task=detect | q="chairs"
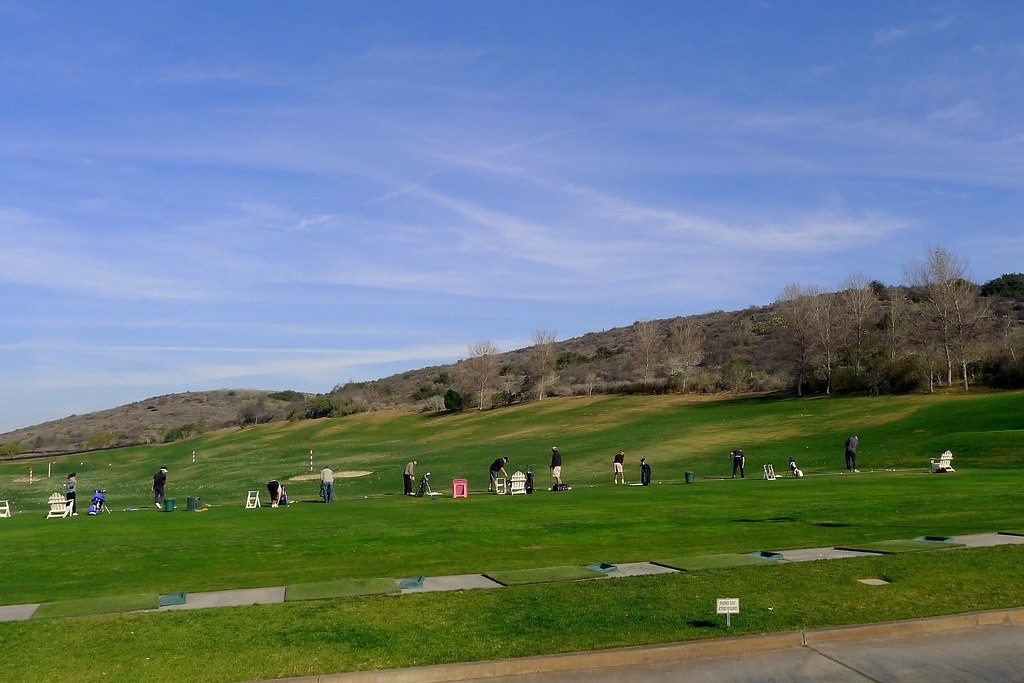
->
[930,450,956,472]
[510,470,527,496]
[763,464,776,481]
[246,490,261,508]
[47,492,74,519]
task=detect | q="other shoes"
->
[271,504,279,508]
[72,512,78,516]
[732,474,736,478]
[155,502,162,509]
[848,470,853,473]
[854,469,860,473]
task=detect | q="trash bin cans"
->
[685,471,694,484]
[164,498,174,511]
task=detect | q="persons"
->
[549,447,562,485]
[490,457,509,492]
[267,480,282,508]
[66,473,78,516]
[845,436,860,472]
[152,467,168,508]
[404,460,418,495]
[730,448,745,478]
[320,467,333,502]
[613,451,625,484]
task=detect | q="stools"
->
[0,500,12,518]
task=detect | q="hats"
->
[620,451,625,455]
[549,446,558,451]
[504,457,510,463]
[855,434,860,443]
[160,467,169,473]
